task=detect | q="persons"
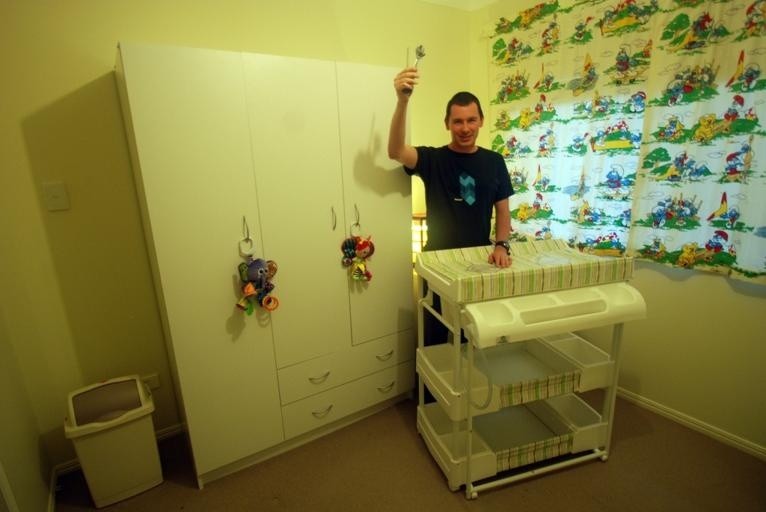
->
[385,66,515,402]
[338,233,377,282]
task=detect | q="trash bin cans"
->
[63,375,164,508]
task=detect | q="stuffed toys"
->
[236,256,279,326]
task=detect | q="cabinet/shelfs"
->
[410,238,649,500]
[113,39,422,494]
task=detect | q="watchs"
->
[495,239,513,256]
